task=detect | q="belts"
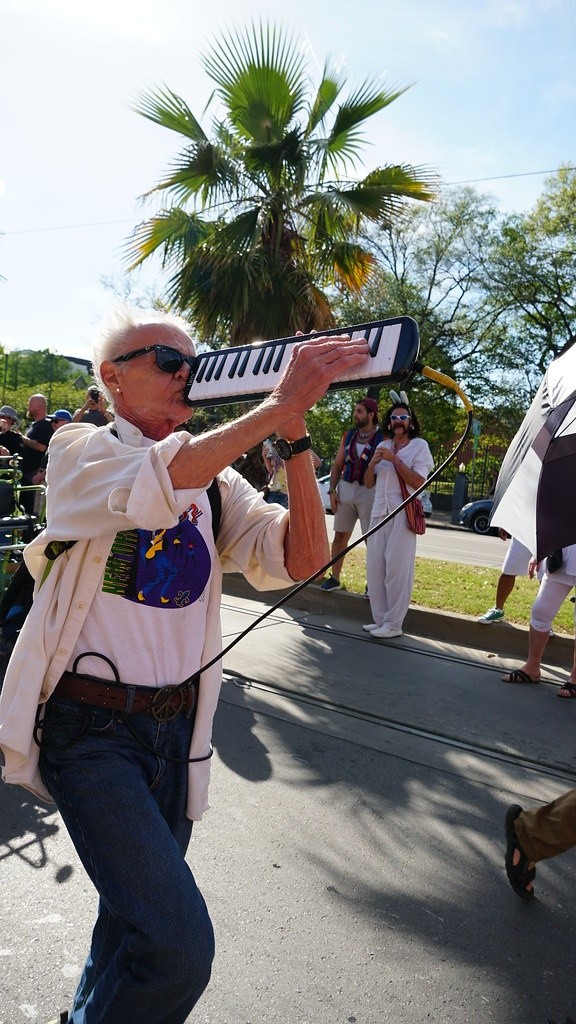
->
[58,676,202,715]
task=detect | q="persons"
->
[33,410,73,512]
[0,308,370,1024]
[500,544,576,697]
[363,389,435,638]
[478,527,554,636]
[15,394,53,514]
[320,399,389,599]
[72,385,116,428]
[0,405,24,456]
[262,448,321,510]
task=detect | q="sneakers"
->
[321,573,341,591]
[370,625,403,638]
[363,585,370,599]
[363,624,381,631]
[477,608,505,624]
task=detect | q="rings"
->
[381,453,382,457]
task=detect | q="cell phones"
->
[91,389,99,403]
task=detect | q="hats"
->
[46,410,73,422]
[360,398,378,414]
[1,406,21,425]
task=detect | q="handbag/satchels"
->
[404,492,425,535]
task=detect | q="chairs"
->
[0,480,38,526]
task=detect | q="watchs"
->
[273,434,312,460]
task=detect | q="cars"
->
[317,472,432,518]
[459,499,510,535]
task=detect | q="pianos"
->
[181,312,422,408]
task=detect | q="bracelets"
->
[327,490,336,495]
[104,413,108,416]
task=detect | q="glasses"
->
[390,415,410,421]
[114,345,196,373]
[51,418,65,424]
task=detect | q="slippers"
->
[506,804,537,898]
[554,681,576,699]
[502,669,542,684]
